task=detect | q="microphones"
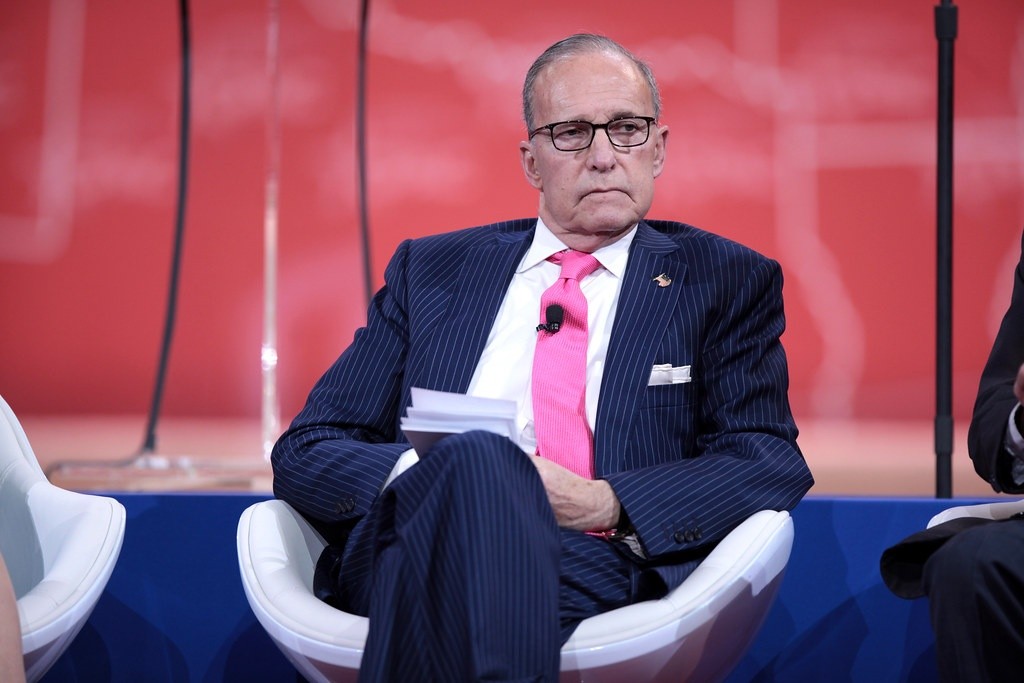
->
[536,305,564,333]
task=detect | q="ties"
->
[530,250,615,540]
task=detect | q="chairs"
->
[0,394,126,683]
[236,498,796,683]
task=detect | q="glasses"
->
[527,116,659,152]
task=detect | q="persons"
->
[271,33,816,683]
[882,231,1024,683]
